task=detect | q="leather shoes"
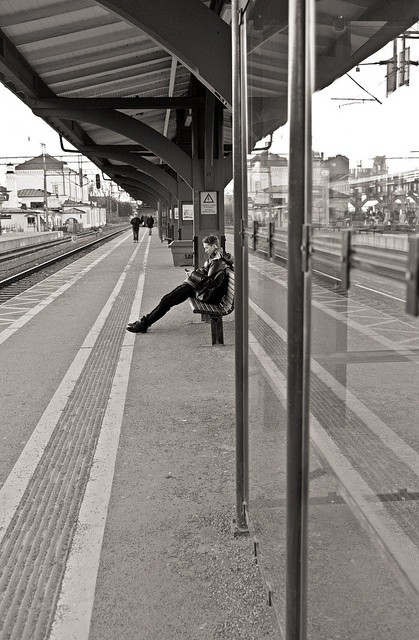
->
[128,321,139,326]
[126,322,147,333]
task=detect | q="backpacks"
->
[195,266,228,308]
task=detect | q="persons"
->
[126,235,229,334]
[130,213,154,243]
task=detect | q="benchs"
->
[187,268,235,346]
